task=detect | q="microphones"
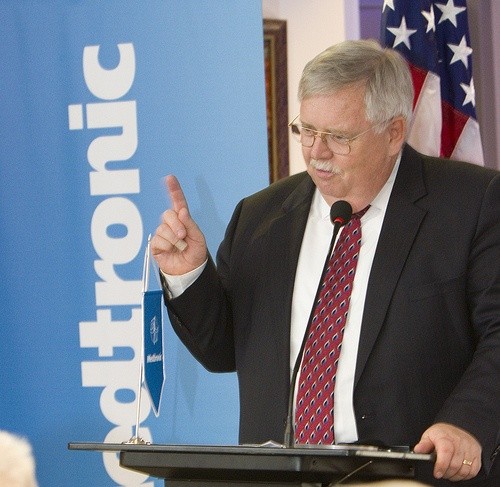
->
[284,200,353,449]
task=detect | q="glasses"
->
[289,113,386,156]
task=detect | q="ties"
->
[294,205,371,445]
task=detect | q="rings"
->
[462,459,473,465]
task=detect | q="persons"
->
[148,39,500,483]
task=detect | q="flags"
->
[143,291,166,418]
[382,0,486,168]
